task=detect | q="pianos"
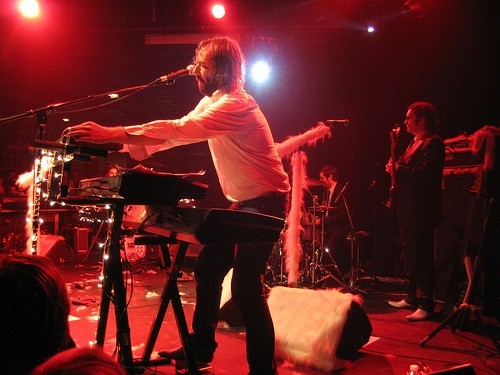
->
[119,200,288,247]
[77,166,210,204]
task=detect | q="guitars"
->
[387,126,403,207]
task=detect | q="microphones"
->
[158,64,195,83]
[334,180,350,204]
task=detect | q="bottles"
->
[407,364,419,375]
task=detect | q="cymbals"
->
[306,175,327,188]
[311,204,338,211]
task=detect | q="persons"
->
[105,165,118,177]
[0,251,127,375]
[306,165,352,293]
[66,38,291,375]
[1,170,32,198]
[385,102,446,321]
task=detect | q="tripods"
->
[418,189,500,354]
[297,194,369,295]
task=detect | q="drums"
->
[301,213,325,242]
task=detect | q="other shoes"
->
[388,299,416,308]
[406,309,433,321]
[158,345,185,360]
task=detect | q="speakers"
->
[22,234,75,265]
[429,166,500,315]
[266,285,372,360]
